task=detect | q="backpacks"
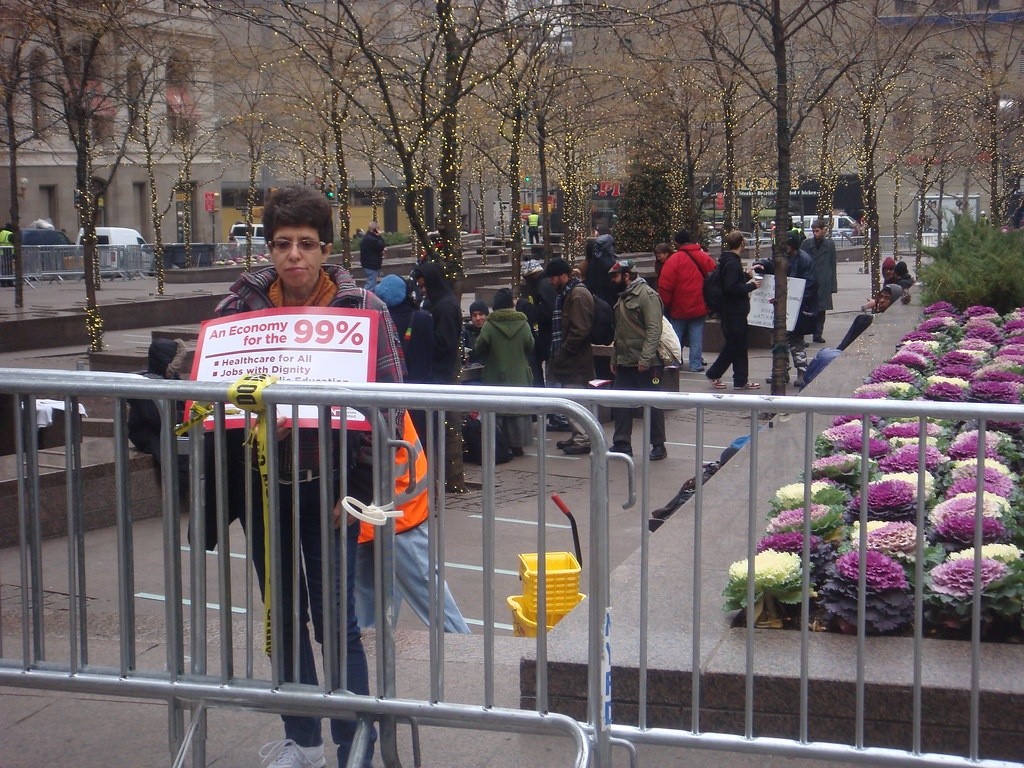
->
[577,285,614,346]
[703,257,739,313]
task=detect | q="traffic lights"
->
[519,176,529,182]
[328,182,335,201]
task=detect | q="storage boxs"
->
[507,552,585,637]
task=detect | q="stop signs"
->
[521,209,532,219]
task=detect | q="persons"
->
[125,338,189,484]
[213,183,407,768]
[351,208,914,462]
[979,211,989,226]
[0,222,20,287]
[353,408,470,635]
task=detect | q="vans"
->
[792,215,863,243]
[76,227,152,274]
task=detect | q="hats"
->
[674,230,691,243]
[788,230,802,249]
[538,257,570,279]
[608,259,638,274]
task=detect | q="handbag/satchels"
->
[658,314,682,366]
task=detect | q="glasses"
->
[270,238,325,252]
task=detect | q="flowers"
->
[725,300,1024,632]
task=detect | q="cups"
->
[755,276,763,282]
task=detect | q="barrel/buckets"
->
[507,593,586,637]
[519,551,581,624]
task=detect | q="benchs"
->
[0,240,873,548]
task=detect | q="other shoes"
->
[734,379,761,390]
[547,421,571,431]
[793,369,806,386]
[555,435,592,455]
[813,334,826,343]
[705,372,726,388]
[607,444,634,458]
[650,445,668,461]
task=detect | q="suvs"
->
[21,229,76,267]
[227,224,266,252]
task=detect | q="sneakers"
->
[267,743,327,768]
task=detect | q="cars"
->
[709,231,771,247]
